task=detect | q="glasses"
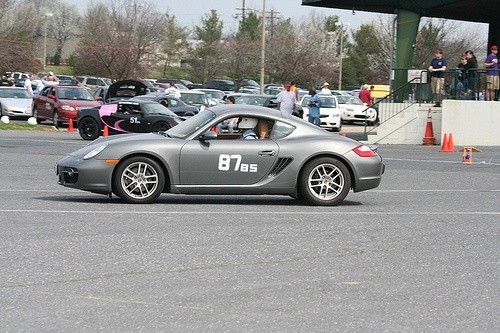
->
[462,58,467,60]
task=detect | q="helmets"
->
[238,118,259,129]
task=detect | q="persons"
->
[309,88,321,126]
[484,45,500,101]
[359,84,375,105]
[321,83,331,94]
[451,50,485,101]
[37,73,59,94]
[25,74,34,95]
[241,120,274,139]
[165,81,181,104]
[277,82,296,115]
[290,81,299,101]
[219,96,238,133]
[0,75,13,86]
[429,49,447,107]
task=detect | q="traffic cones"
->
[67,119,75,133]
[102,125,109,137]
[446,133,456,152]
[422,108,436,146]
[439,133,449,153]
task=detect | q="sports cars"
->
[54,102,385,206]
[76,98,188,141]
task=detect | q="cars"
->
[0,70,392,140]
[30,84,101,127]
[0,85,35,119]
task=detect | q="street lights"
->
[335,17,350,91]
[43,12,54,72]
